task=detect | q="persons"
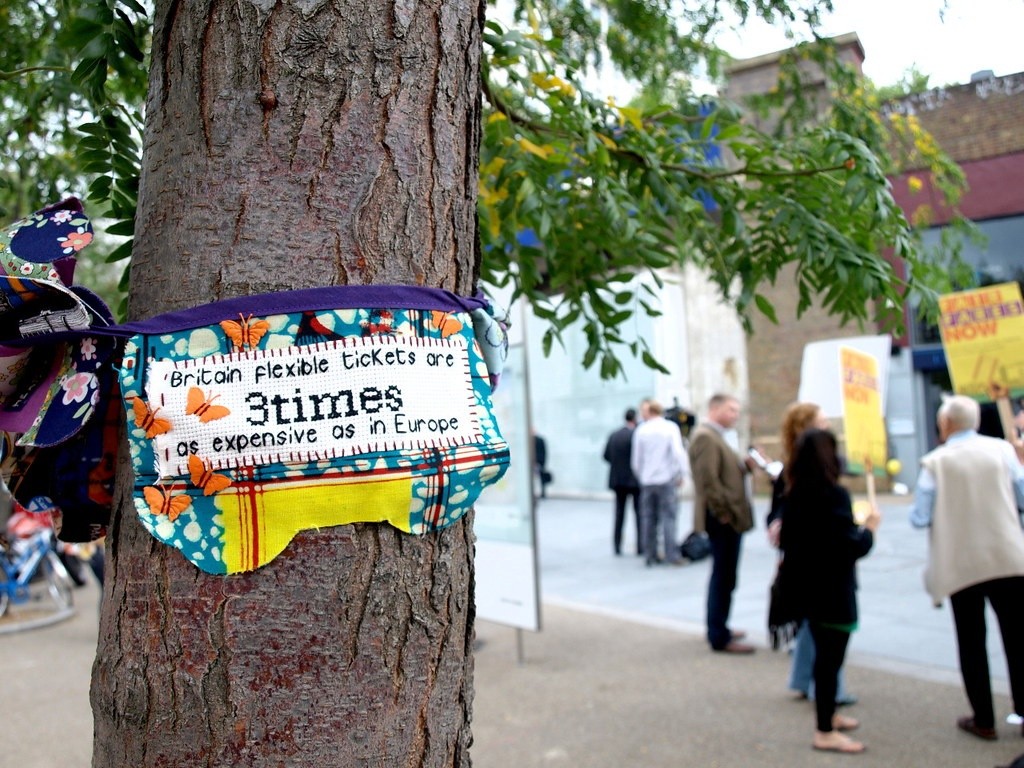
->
[637,397,651,424]
[533,431,546,498]
[631,402,690,567]
[773,405,856,707]
[909,396,1024,738]
[690,395,760,654]
[780,429,878,755]
[604,409,641,555]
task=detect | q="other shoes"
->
[647,557,666,565]
[831,713,859,729]
[813,730,867,753]
[835,693,857,705]
[672,556,690,565]
[728,629,746,639]
[723,641,753,653]
[960,715,998,739]
